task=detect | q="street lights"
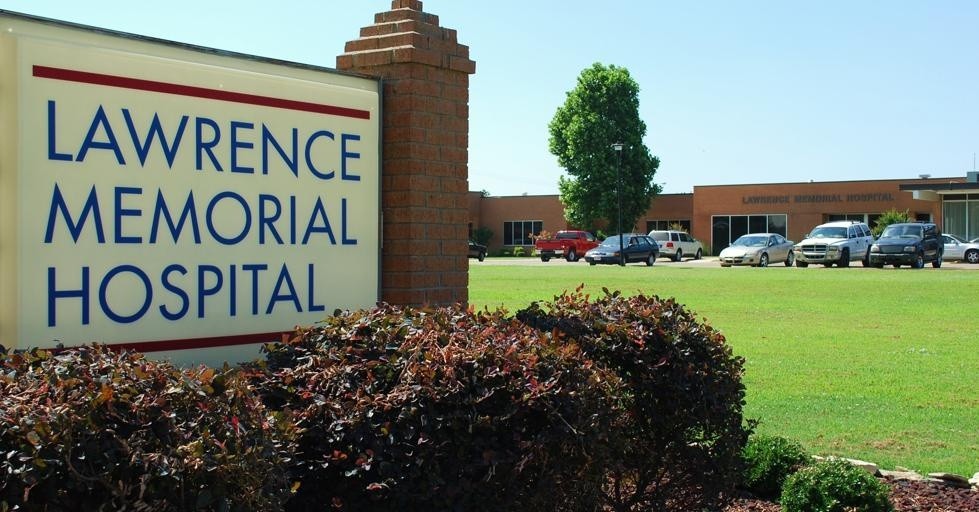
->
[612,140,625,266]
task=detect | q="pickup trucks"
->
[534,230,603,262]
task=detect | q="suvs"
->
[793,220,944,267]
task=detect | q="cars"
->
[584,230,703,266]
[942,220,979,268]
[719,232,795,267]
[468,237,488,261]
